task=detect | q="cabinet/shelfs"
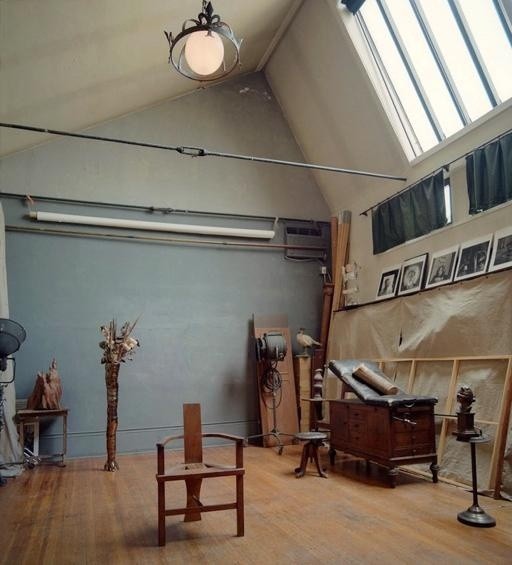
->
[326,397,439,488]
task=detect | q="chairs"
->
[156,402,245,547]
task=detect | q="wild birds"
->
[296,328,322,354]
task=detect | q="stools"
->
[295,432,328,479]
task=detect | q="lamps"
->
[162,0,245,90]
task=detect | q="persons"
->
[498,241,512,261]
[431,266,449,282]
[404,266,420,289]
[383,279,393,295]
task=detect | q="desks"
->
[16,408,68,468]
